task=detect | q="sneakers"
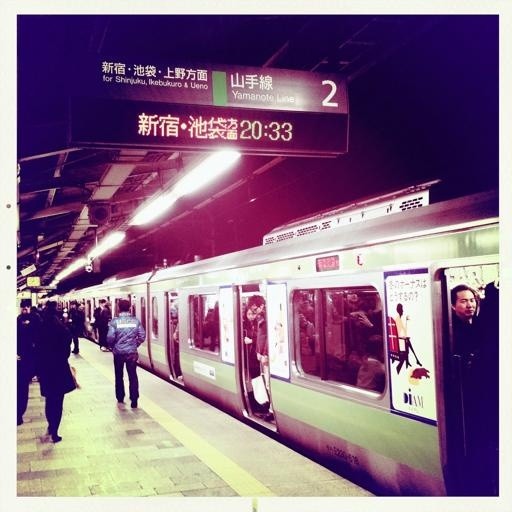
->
[264,414,274,423]
[72,348,79,354]
[116,396,138,409]
[52,434,62,443]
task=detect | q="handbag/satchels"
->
[251,372,269,405]
[63,363,80,394]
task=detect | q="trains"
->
[37,179,499,496]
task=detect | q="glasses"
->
[252,306,260,313]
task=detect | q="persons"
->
[17,298,86,441]
[447,281,499,472]
[171,304,183,380]
[241,295,275,422]
[193,298,219,352]
[92,299,146,409]
[297,286,385,394]
